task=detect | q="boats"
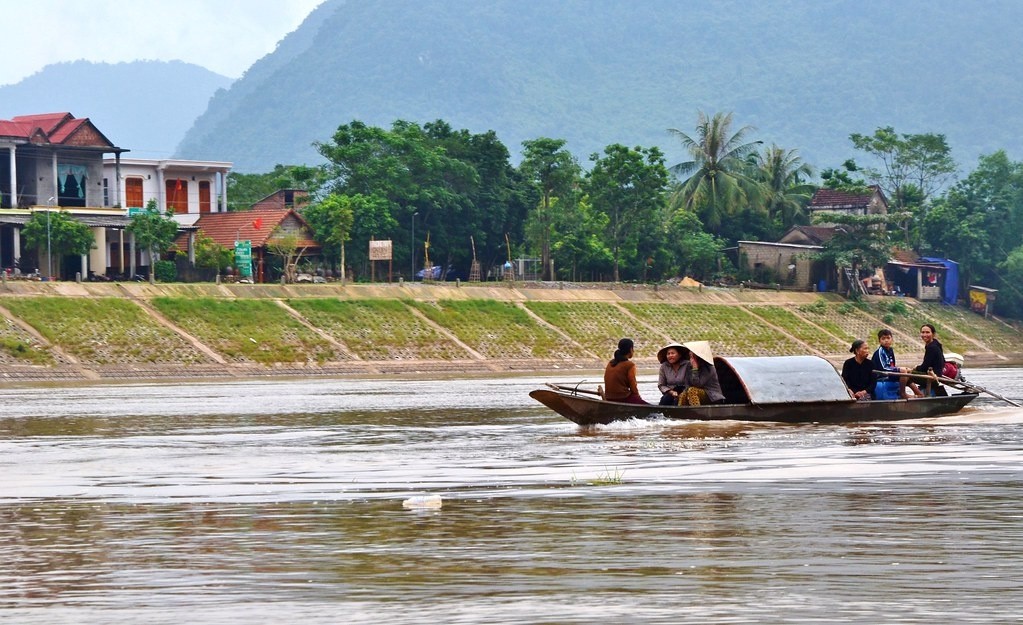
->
[528,355,981,424]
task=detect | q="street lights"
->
[412,211,419,282]
[47,196,55,281]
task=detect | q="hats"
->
[657,341,691,364]
[683,340,715,366]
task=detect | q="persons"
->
[842,341,878,401]
[603,339,649,403]
[872,330,925,398]
[911,324,945,388]
[658,341,723,407]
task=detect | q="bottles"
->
[402,494,442,509]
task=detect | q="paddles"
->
[545,382,600,396]
[872,369,965,385]
[942,374,1021,407]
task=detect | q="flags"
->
[175,177,181,190]
